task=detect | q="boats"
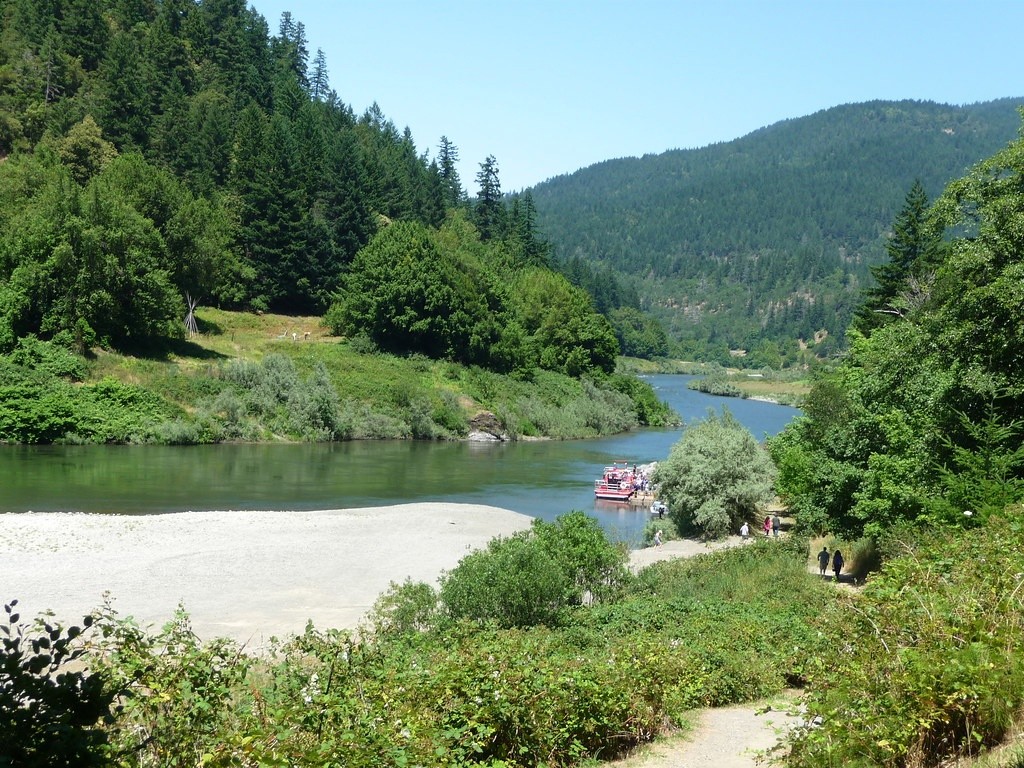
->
[594,461,642,499]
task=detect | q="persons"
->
[763,516,770,535]
[832,550,844,579]
[659,508,664,519]
[740,522,749,540]
[772,514,780,535]
[653,530,662,549]
[817,547,829,578]
[633,464,636,474]
[643,478,648,490]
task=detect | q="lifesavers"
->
[620,482,626,489]
